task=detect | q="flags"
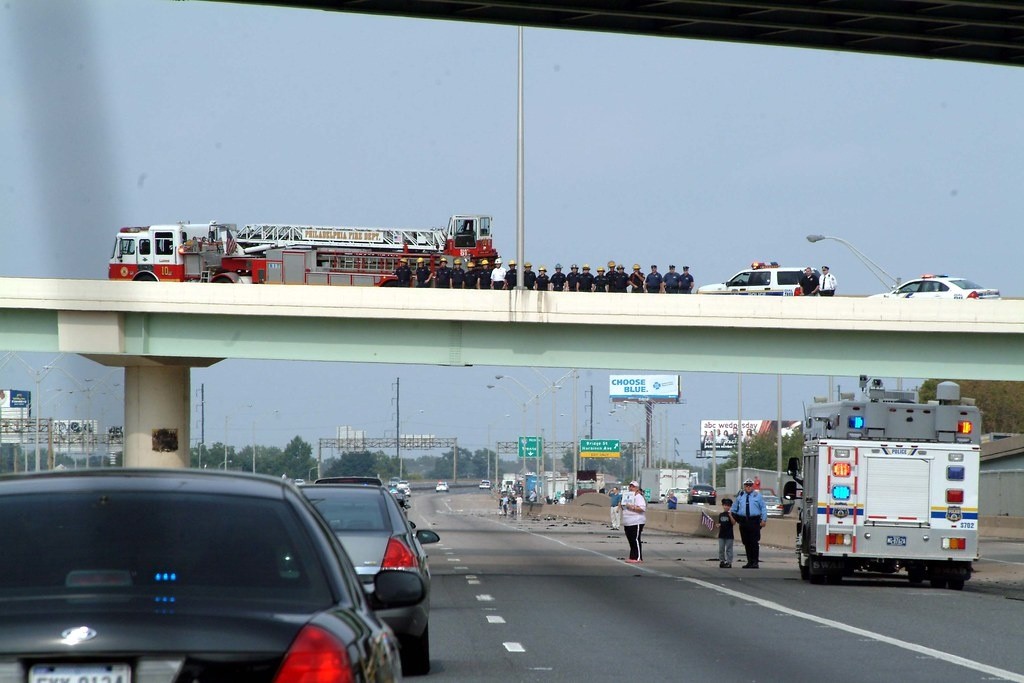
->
[701,513,714,531]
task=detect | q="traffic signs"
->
[518,436,543,459]
[579,439,621,458]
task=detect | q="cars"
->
[479,480,491,491]
[0,468,428,683]
[733,487,795,518]
[864,273,1001,299]
[285,482,441,678]
[658,485,718,505]
[435,482,450,492]
[386,476,413,520]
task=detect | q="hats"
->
[822,266,829,270]
[651,265,690,269]
[630,481,639,487]
[743,478,754,485]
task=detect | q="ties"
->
[823,278,825,289]
[746,494,749,519]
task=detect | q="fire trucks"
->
[108,215,499,288]
[783,375,983,589]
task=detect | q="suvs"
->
[696,262,822,297]
[311,473,386,530]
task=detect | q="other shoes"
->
[720,561,731,568]
[625,559,638,563]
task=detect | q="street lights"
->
[308,466,318,484]
[487,384,530,507]
[224,400,254,471]
[218,459,232,470]
[494,374,545,505]
[0,349,125,472]
[199,440,216,469]
[252,409,280,473]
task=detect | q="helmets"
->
[509,260,516,266]
[597,267,606,272]
[571,264,578,269]
[467,262,475,268]
[494,259,502,264]
[634,264,641,269]
[608,262,616,267]
[617,265,625,269]
[538,267,545,271]
[583,264,590,270]
[416,258,424,263]
[440,258,448,264]
[524,263,532,267]
[555,264,561,268]
[400,258,408,263]
[481,260,489,264]
[454,260,462,265]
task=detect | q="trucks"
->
[639,467,690,503]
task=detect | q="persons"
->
[434,258,518,290]
[512,495,523,514]
[667,492,677,509]
[396,258,413,287]
[416,257,433,288]
[800,268,819,296]
[716,498,736,569]
[730,478,767,569]
[529,491,537,502]
[819,266,837,296]
[500,496,509,514]
[524,263,695,294]
[618,481,647,563]
[608,487,622,530]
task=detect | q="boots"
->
[742,551,759,568]
